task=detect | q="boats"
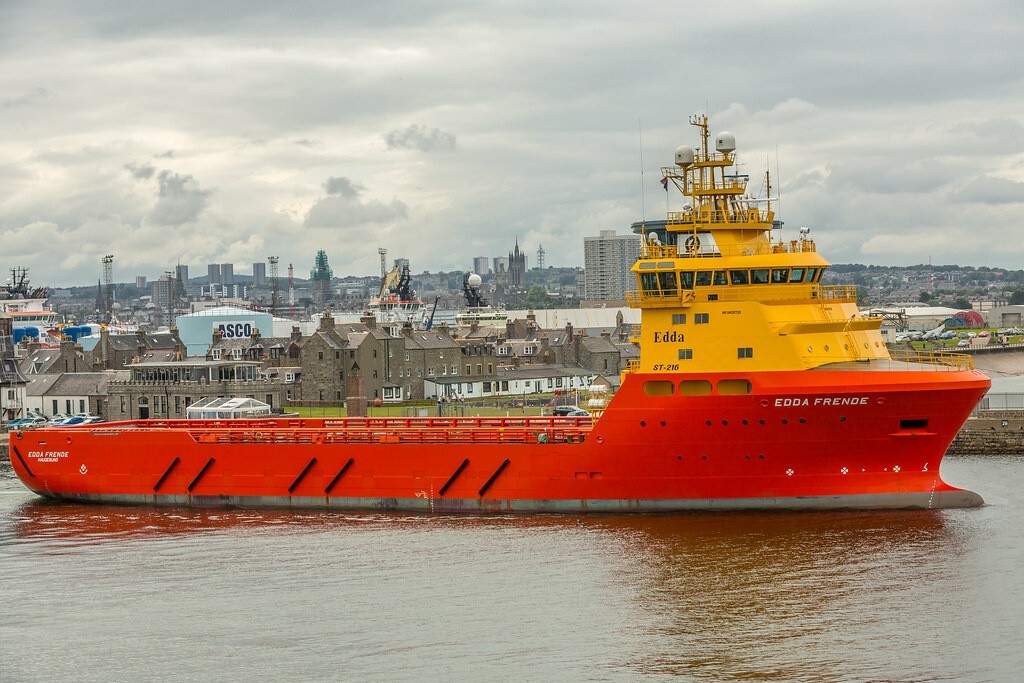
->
[8,112,992,515]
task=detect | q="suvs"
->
[553,405,580,416]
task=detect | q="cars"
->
[567,410,589,416]
[8,411,101,431]
[895,328,1024,348]
